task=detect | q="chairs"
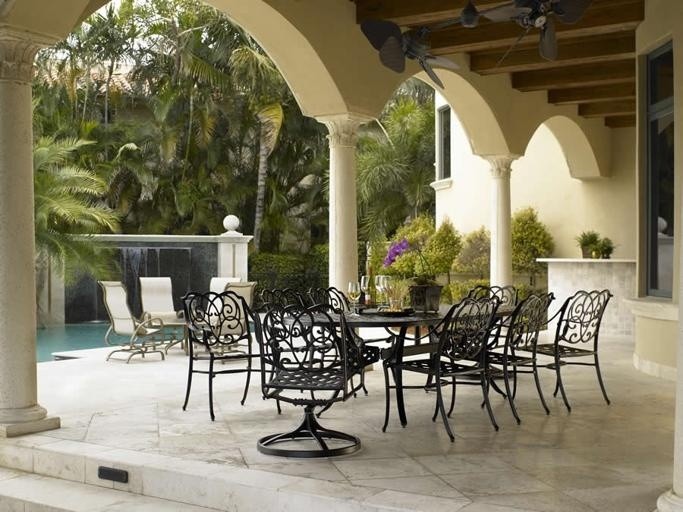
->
[97,276,259,365]
[511,289,613,414]
[182,291,263,420]
[257,285,553,457]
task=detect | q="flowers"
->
[381,236,436,284]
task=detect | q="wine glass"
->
[346,280,361,319]
[360,276,395,306]
